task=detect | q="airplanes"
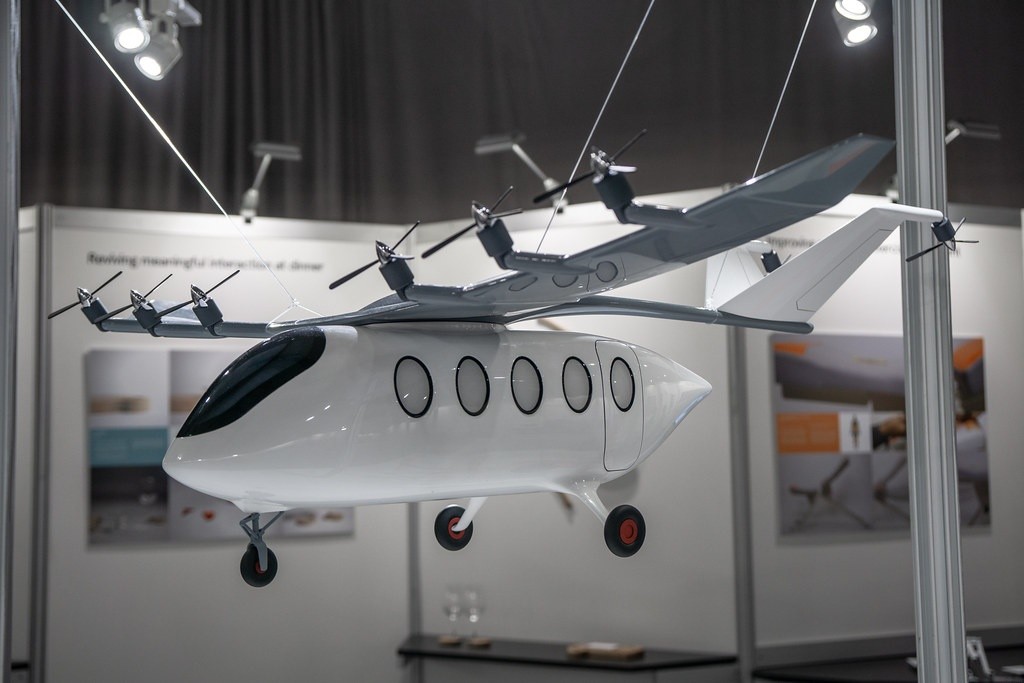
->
[47,126,977,588]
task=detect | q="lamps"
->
[830,0,878,47]
[99,0,203,80]
